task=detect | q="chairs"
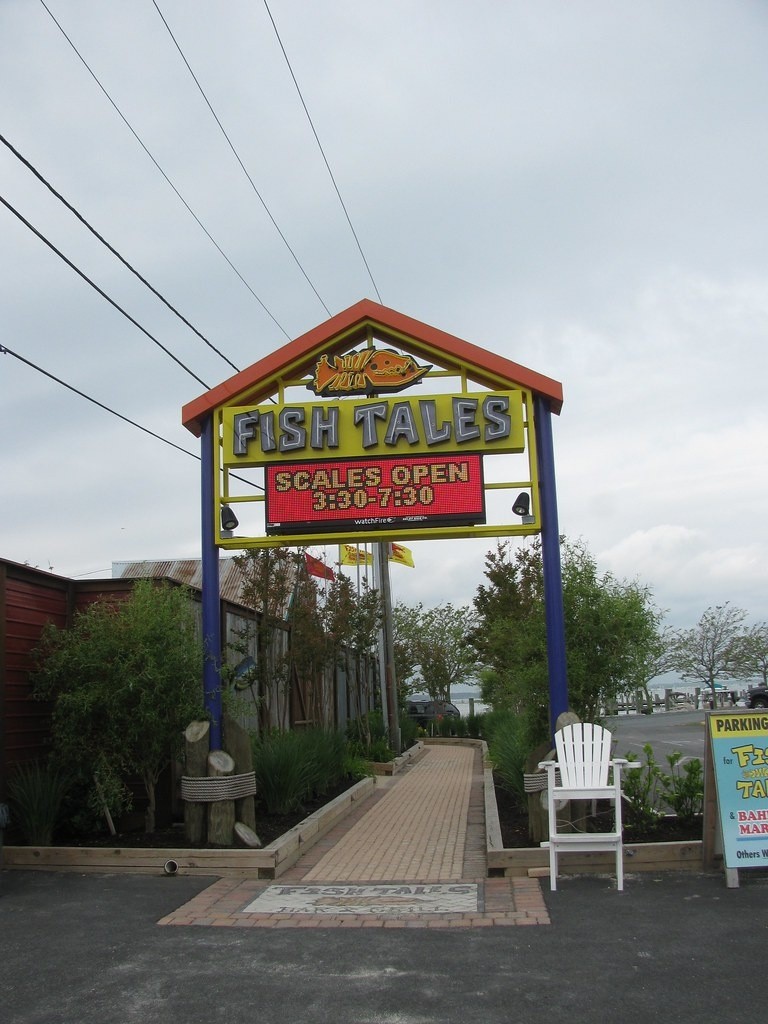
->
[538,723,622,892]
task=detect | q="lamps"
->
[232,656,258,690]
[511,492,533,519]
[220,506,239,530]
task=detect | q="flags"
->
[306,553,335,582]
[340,544,372,566]
[387,542,414,568]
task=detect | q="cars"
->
[405,700,460,732]
[745,686,768,711]
